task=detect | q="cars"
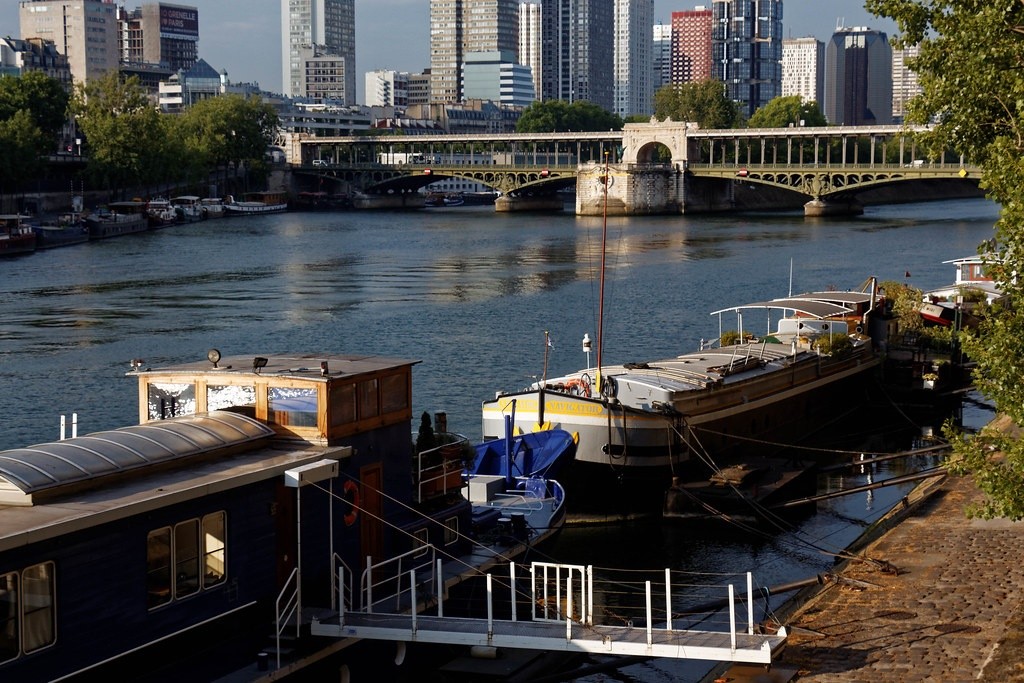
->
[904,160,930,168]
[428,155,441,164]
[303,160,312,166]
[409,156,430,164]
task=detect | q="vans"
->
[313,160,328,167]
[23,197,64,215]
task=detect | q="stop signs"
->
[542,169,548,176]
[424,168,431,175]
[739,168,748,176]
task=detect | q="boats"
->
[481,256,896,498]
[225,191,288,211]
[298,192,329,210]
[0,349,581,683]
[146,197,178,225]
[425,194,464,207]
[202,198,227,218]
[169,196,208,224]
[88,201,148,238]
[32,212,90,243]
[0,213,37,255]
[916,252,1018,310]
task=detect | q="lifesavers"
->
[344,480,358,524]
[562,378,592,403]
